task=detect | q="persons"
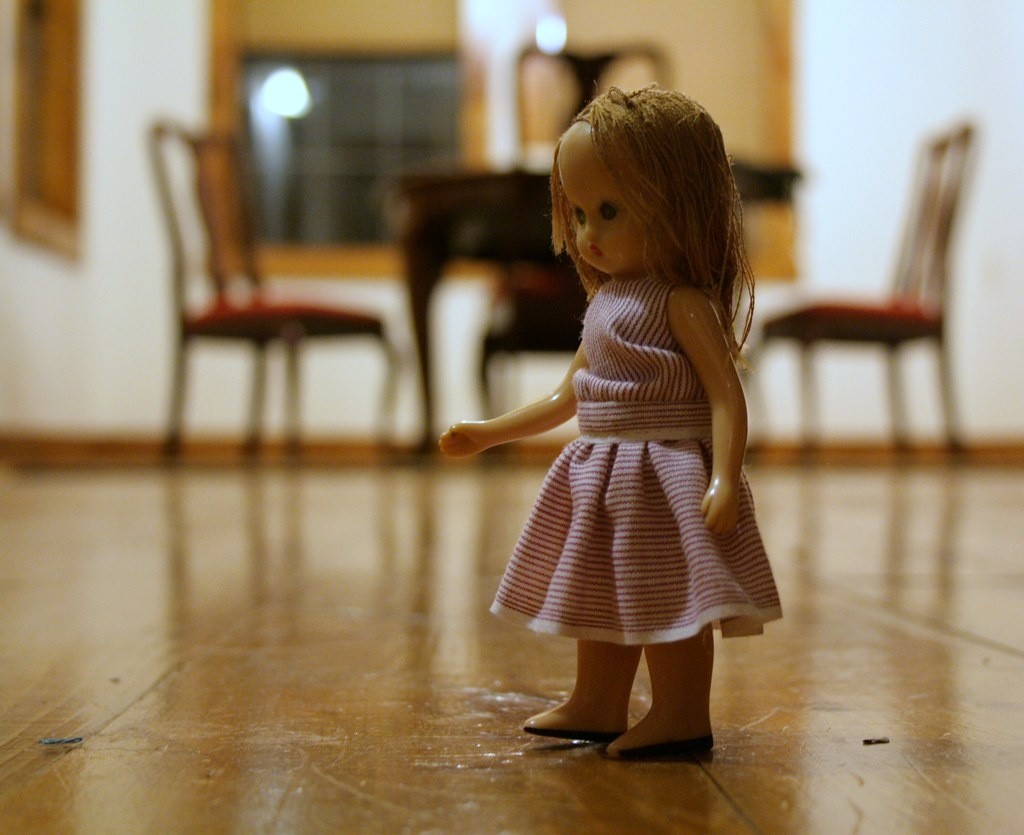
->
[437,88,785,761]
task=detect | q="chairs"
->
[742,124,975,469]
[150,121,405,468]
[473,42,668,422]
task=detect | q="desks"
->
[393,167,590,451]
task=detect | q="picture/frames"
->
[8,1,86,259]
